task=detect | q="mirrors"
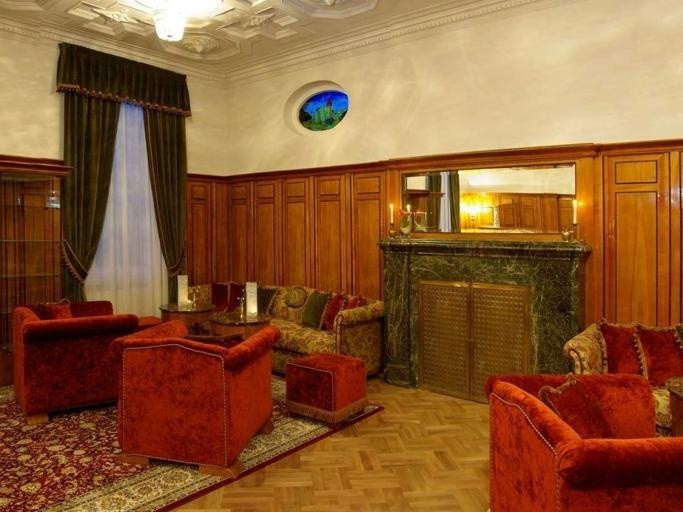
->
[401,162,577,236]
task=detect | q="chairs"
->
[480,316,683,511]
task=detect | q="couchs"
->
[183,278,383,381]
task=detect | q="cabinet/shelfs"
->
[495,191,573,234]
[404,188,445,233]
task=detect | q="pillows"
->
[207,279,278,336]
[299,288,366,334]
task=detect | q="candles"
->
[388,203,395,223]
[572,198,578,226]
[406,201,412,224]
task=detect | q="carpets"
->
[0,375,385,512]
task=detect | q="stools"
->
[283,348,369,428]
